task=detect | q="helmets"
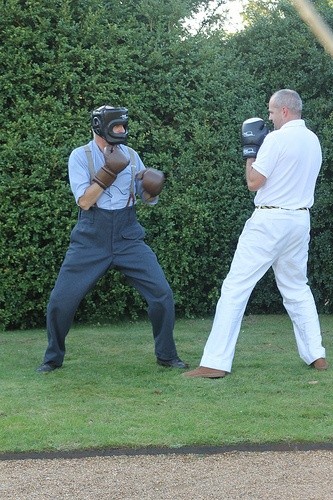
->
[91,108,129,145]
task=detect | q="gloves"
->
[240,117,266,158]
[92,148,129,191]
[137,167,165,195]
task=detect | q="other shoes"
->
[155,360,187,370]
[313,359,328,370]
[178,367,226,379]
[36,363,54,372]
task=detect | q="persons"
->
[35,105,190,372]
[178,89,326,377]
